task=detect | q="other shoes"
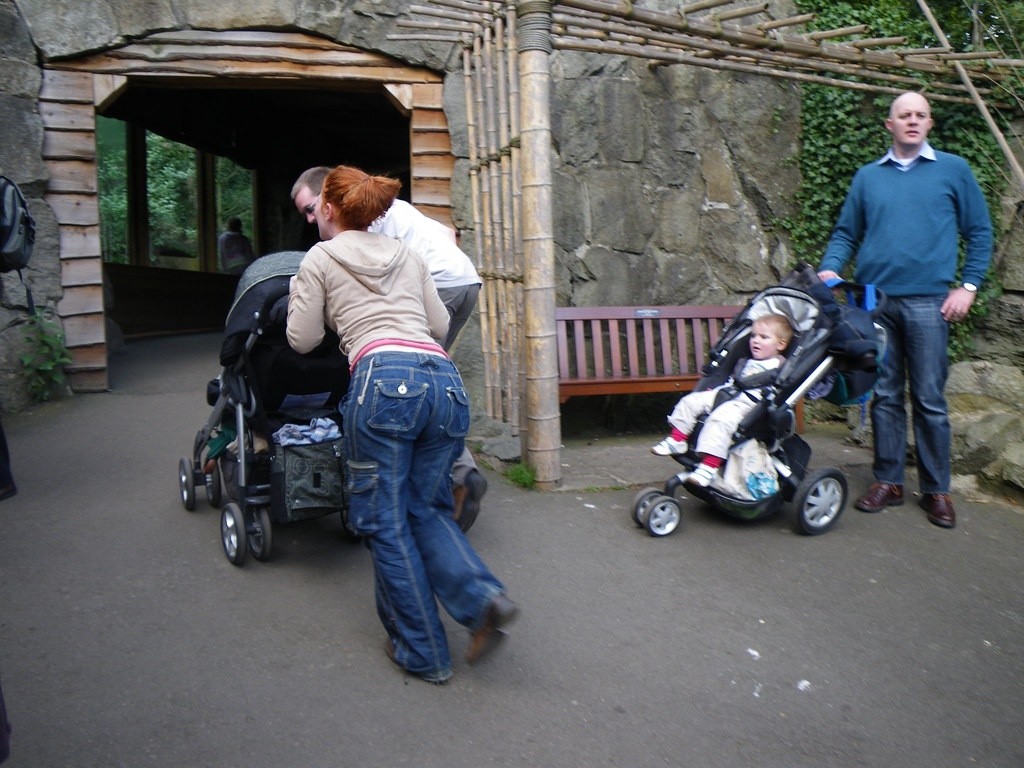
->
[452,469,487,535]
[466,594,516,665]
[0,480,16,501]
[385,635,399,663]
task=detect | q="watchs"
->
[962,282,977,291]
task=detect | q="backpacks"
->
[0,176,36,273]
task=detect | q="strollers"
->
[180,254,365,568]
[631,265,889,540]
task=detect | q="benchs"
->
[554,304,804,434]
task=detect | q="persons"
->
[649,313,794,487]
[219,164,523,685]
[0,422,19,502]
[816,89,992,528]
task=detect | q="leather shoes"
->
[854,482,904,512]
[918,492,956,527]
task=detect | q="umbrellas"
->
[202,417,237,474]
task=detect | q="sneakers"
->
[650,435,688,456]
[687,462,720,488]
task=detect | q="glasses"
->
[302,193,321,218]
